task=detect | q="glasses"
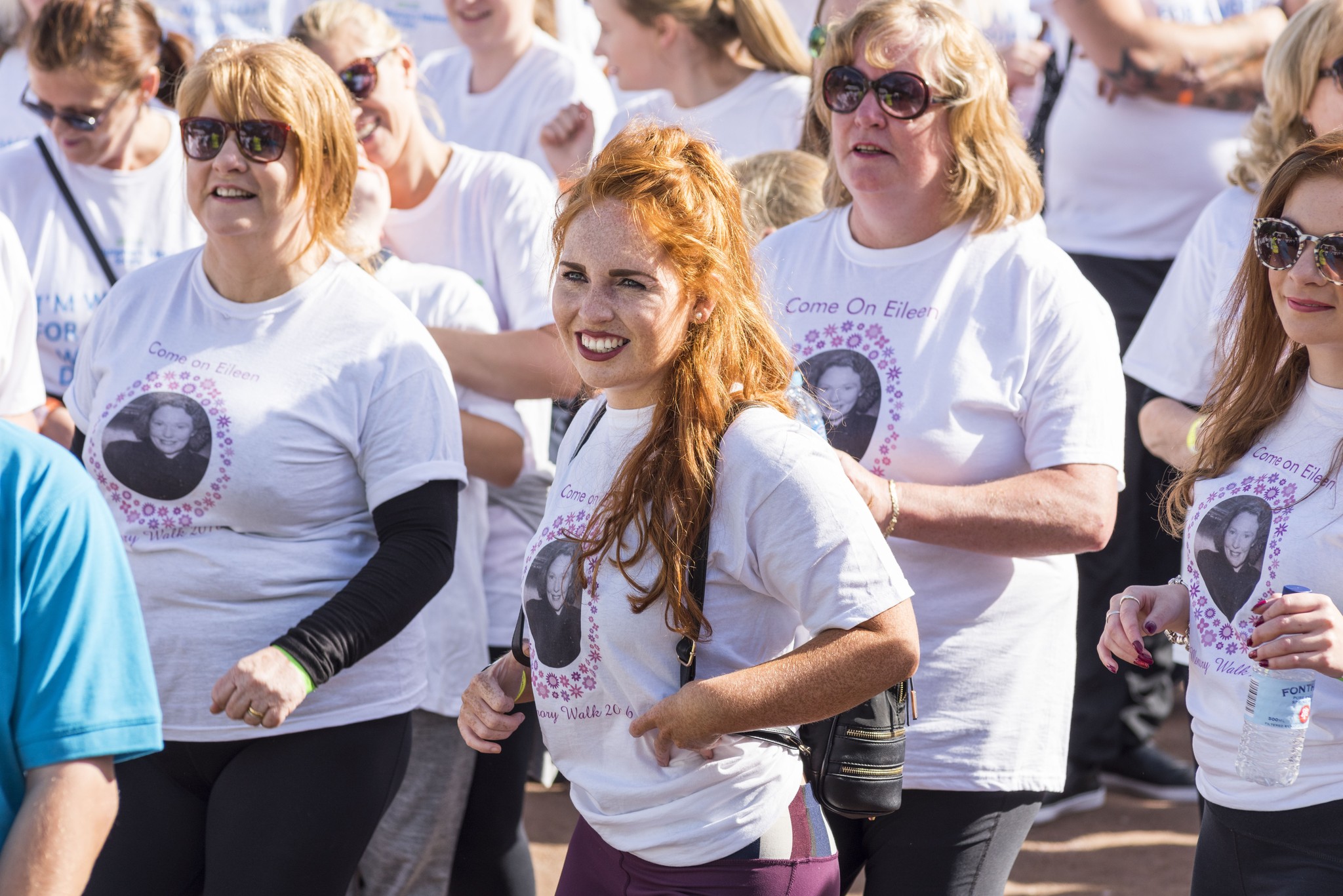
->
[1251,217,1343,287]
[19,77,128,131]
[822,65,956,120]
[337,46,395,101]
[1319,54,1343,95]
[179,118,293,164]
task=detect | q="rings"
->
[247,707,263,718]
[1106,611,1120,623]
[1120,595,1140,607]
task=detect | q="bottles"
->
[1232,585,1317,789]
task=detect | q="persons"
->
[61,37,468,896]
[0,0,1343,896]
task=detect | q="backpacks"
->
[510,397,917,819]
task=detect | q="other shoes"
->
[1099,744,1201,801]
[1032,774,1108,827]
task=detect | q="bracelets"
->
[481,658,526,702]
[883,479,900,540]
[1164,575,1190,651]
[1177,90,1194,104]
